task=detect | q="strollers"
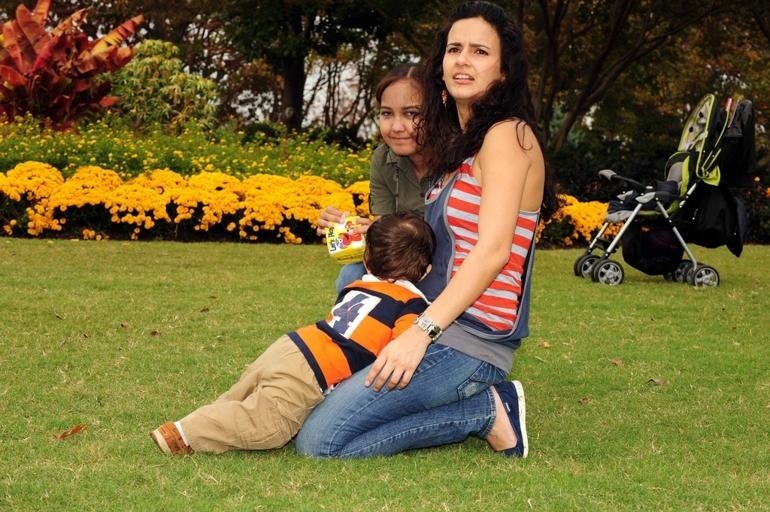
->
[574,87,758,289]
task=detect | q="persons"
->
[315,59,443,288]
[293,4,543,459]
[147,202,435,457]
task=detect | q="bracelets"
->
[413,313,442,343]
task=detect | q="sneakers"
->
[149,420,192,456]
[491,379,530,459]
